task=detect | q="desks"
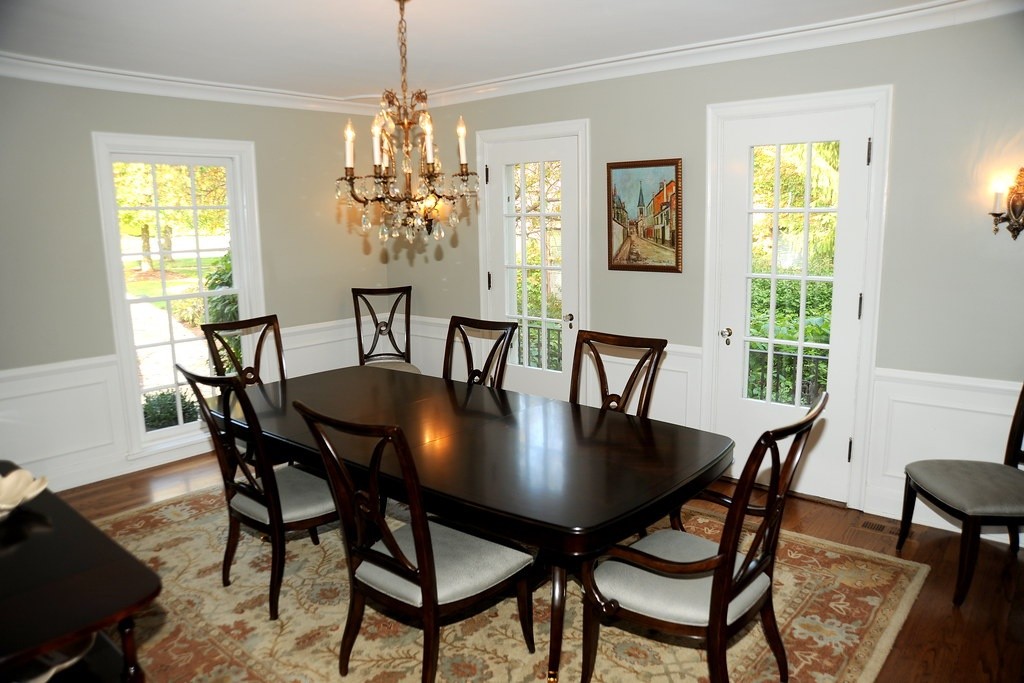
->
[0,459,162,682]
[198,365,736,683]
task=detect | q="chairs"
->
[569,400,661,458]
[446,377,519,428]
[351,285,421,374]
[293,398,537,683]
[579,391,829,682]
[442,315,518,390]
[568,329,669,417]
[217,379,286,423]
[894,383,1024,608]
[200,313,286,396]
[174,361,340,620]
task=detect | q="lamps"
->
[336,0,479,245]
[987,166,1024,241]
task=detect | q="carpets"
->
[91,458,930,683]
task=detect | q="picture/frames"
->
[606,157,684,273]
